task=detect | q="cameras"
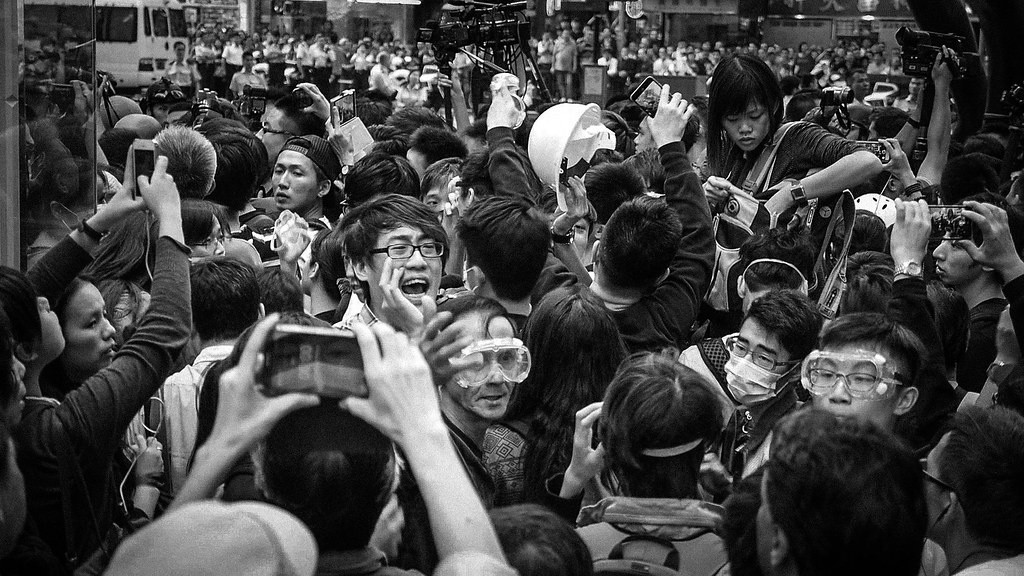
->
[248,87,268,133]
[261,323,368,400]
[820,86,854,107]
[330,89,357,128]
[48,84,74,110]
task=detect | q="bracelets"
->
[908,117,920,130]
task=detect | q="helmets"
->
[143,77,186,106]
[527,103,615,213]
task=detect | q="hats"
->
[279,134,341,182]
[103,501,317,576]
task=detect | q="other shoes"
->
[553,97,572,103]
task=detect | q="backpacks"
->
[574,519,730,576]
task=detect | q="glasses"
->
[371,241,446,260]
[454,340,533,386]
[261,120,293,135]
[799,350,905,402]
[728,336,800,371]
[917,458,954,494]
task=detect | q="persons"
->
[942,208,962,237]
[0,0,1024,576]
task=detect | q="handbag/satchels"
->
[704,121,857,315]
[64,528,112,576]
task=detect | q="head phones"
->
[736,260,808,298]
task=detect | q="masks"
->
[725,354,799,404]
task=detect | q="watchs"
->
[790,180,808,207]
[893,257,922,278]
[78,217,109,245]
[550,226,575,246]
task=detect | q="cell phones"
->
[926,204,973,241]
[630,76,674,118]
[855,140,893,165]
[292,88,312,109]
[134,139,157,201]
